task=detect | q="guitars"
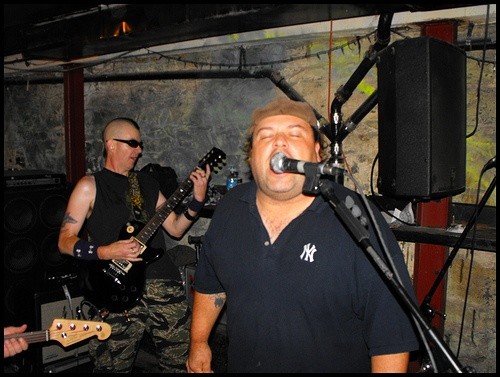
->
[79,146,227,313]
[4,319,112,349]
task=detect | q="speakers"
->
[0,185,76,321]
[375,35,466,200]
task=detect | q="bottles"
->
[227,164,239,190]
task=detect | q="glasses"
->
[106,139,143,150]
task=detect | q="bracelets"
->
[184,211,199,221]
[188,198,205,212]
[74,241,102,261]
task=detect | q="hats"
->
[248,96,319,140]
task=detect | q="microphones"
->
[270,152,347,175]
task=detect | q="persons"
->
[58,118,211,372]
[185,99,419,373]
